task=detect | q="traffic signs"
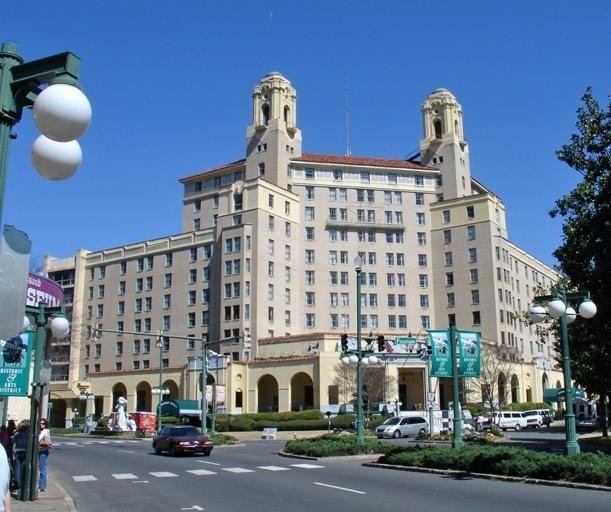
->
[396,337,416,345]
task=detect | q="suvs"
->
[376,416,430,438]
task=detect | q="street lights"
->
[74,408,78,425]
[528,279,597,457]
[354,255,365,446]
[79,392,94,421]
[0,42,92,218]
[19,301,72,499]
[152,385,170,433]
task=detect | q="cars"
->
[151,426,213,457]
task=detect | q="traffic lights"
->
[378,333,386,351]
[342,334,348,352]
[242,330,252,354]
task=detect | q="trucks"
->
[94,413,157,438]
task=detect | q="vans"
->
[490,412,527,431]
[527,409,551,429]
[571,396,597,428]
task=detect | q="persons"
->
[1,415,54,497]
